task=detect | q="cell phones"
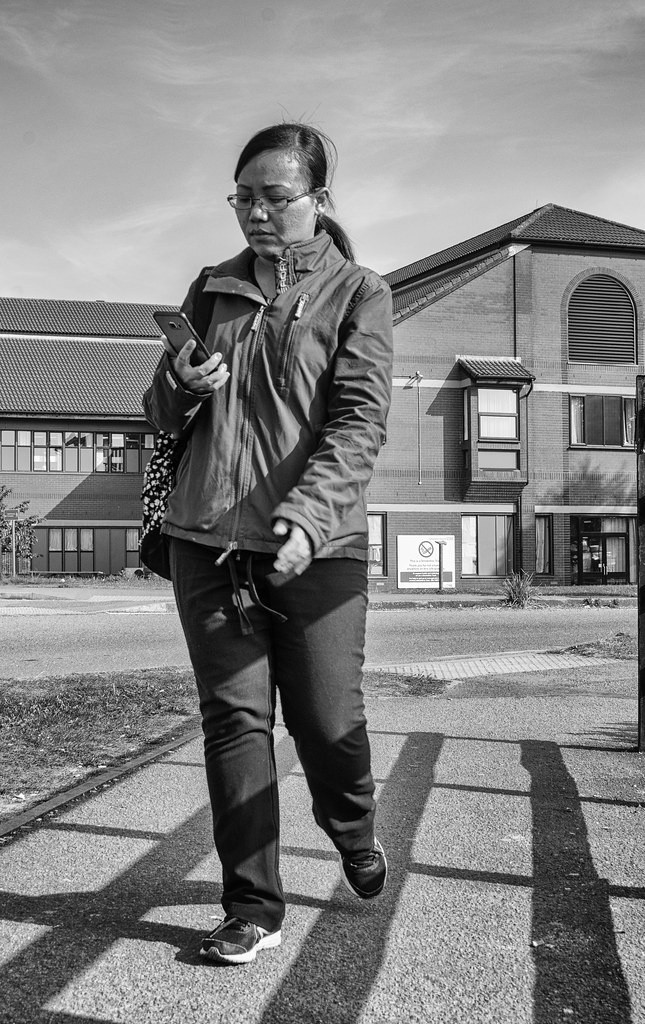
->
[153,310,218,376]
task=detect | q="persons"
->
[141,124,393,967]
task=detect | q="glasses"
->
[227,189,316,211]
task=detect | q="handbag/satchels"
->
[139,266,217,582]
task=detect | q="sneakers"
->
[340,835,388,898]
[200,916,281,963]
